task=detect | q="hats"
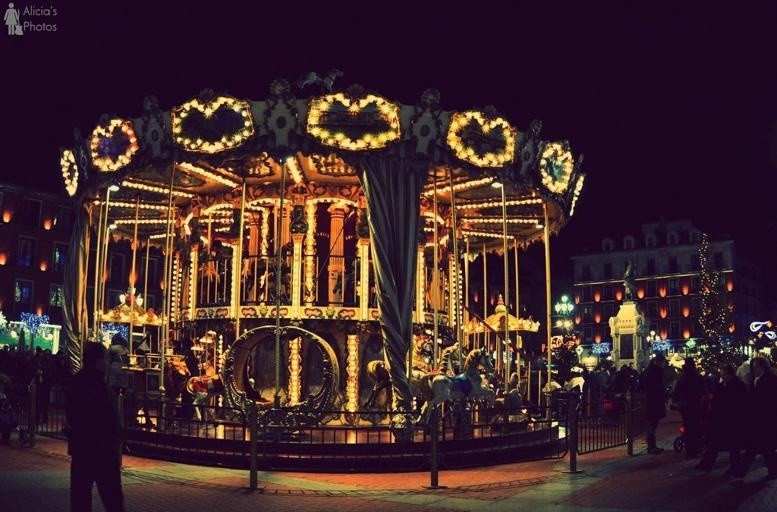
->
[82,341,107,367]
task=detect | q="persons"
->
[510,347,777,488]
[0,331,212,511]
